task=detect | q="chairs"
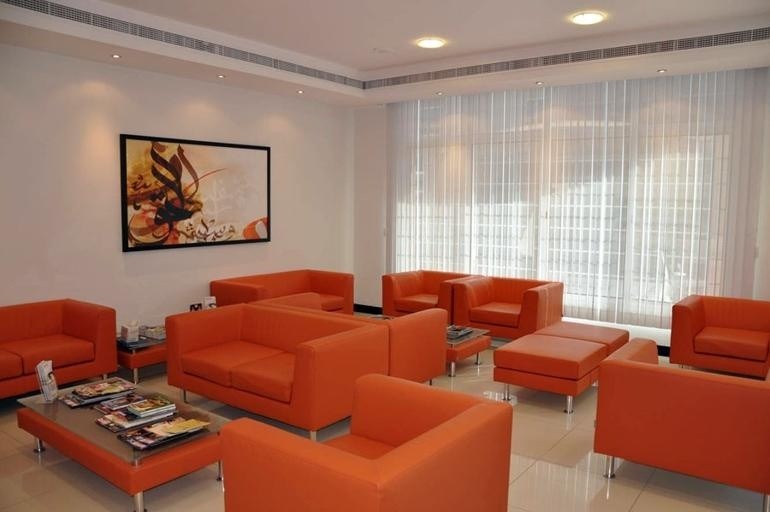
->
[594,337,770,512]
[382,270,482,324]
[670,294,770,378]
[453,276,563,340]
[221,373,512,512]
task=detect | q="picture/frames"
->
[120,135,270,252]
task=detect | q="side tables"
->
[118,331,167,385]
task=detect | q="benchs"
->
[493,320,629,414]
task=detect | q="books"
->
[58,376,210,451]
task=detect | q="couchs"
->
[165,303,389,442]
[0,297,118,400]
[248,292,448,385]
[210,270,354,316]
